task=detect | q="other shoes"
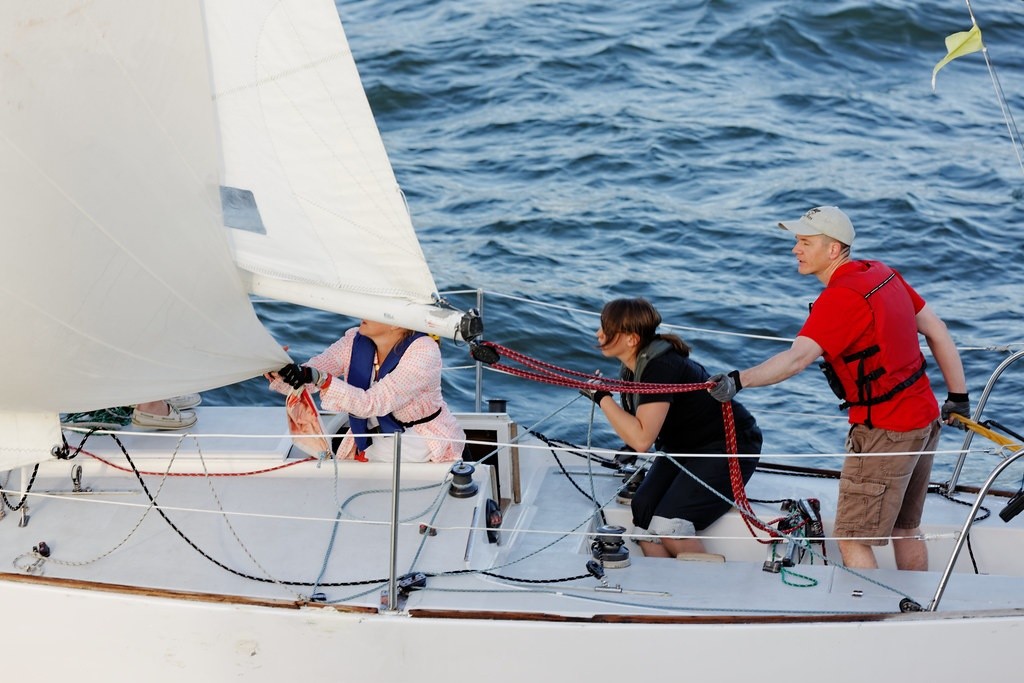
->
[132,403,198,429]
[170,394,202,409]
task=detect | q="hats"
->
[778,206,855,246]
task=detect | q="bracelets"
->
[315,370,332,389]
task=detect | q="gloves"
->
[941,392,971,432]
[613,446,637,467]
[708,370,742,404]
[278,363,320,391]
[579,378,613,407]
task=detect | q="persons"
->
[265,319,467,463]
[586,299,763,558]
[708,205,971,571]
[131,393,202,429]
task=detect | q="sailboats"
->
[2,0,1024,683]
